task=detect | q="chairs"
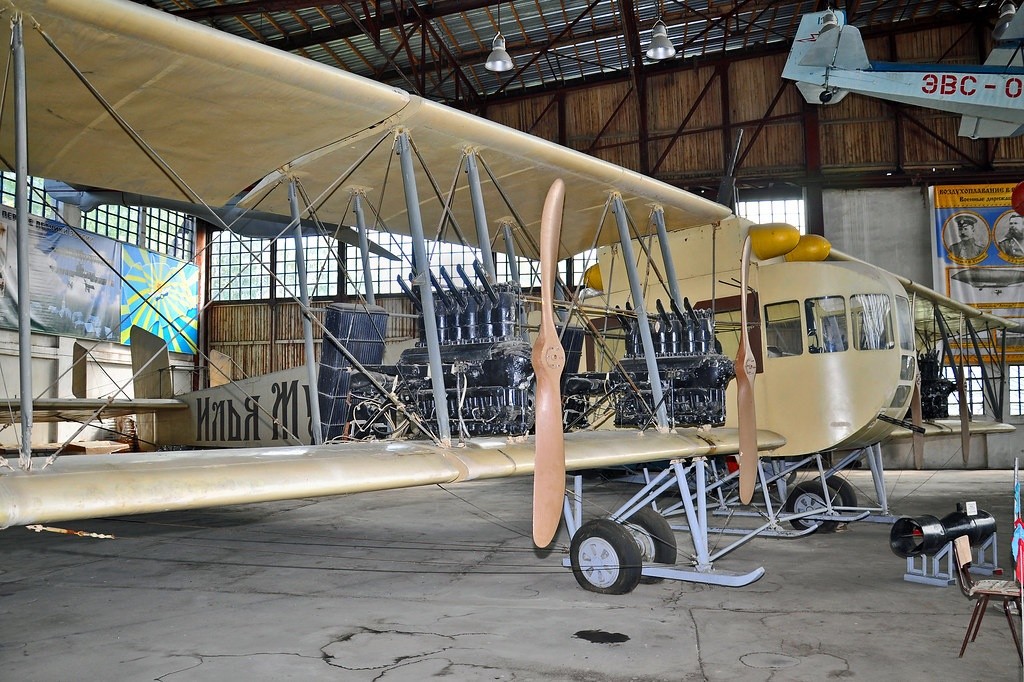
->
[767,345,783,358]
[954,535,1023,667]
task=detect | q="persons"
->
[947,215,986,261]
[997,212,1024,256]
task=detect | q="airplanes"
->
[0,0,1024,596]
[780,3,1024,140]
[48,247,116,293]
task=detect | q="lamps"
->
[992,0,1020,40]
[646,20,676,60]
[485,32,513,71]
